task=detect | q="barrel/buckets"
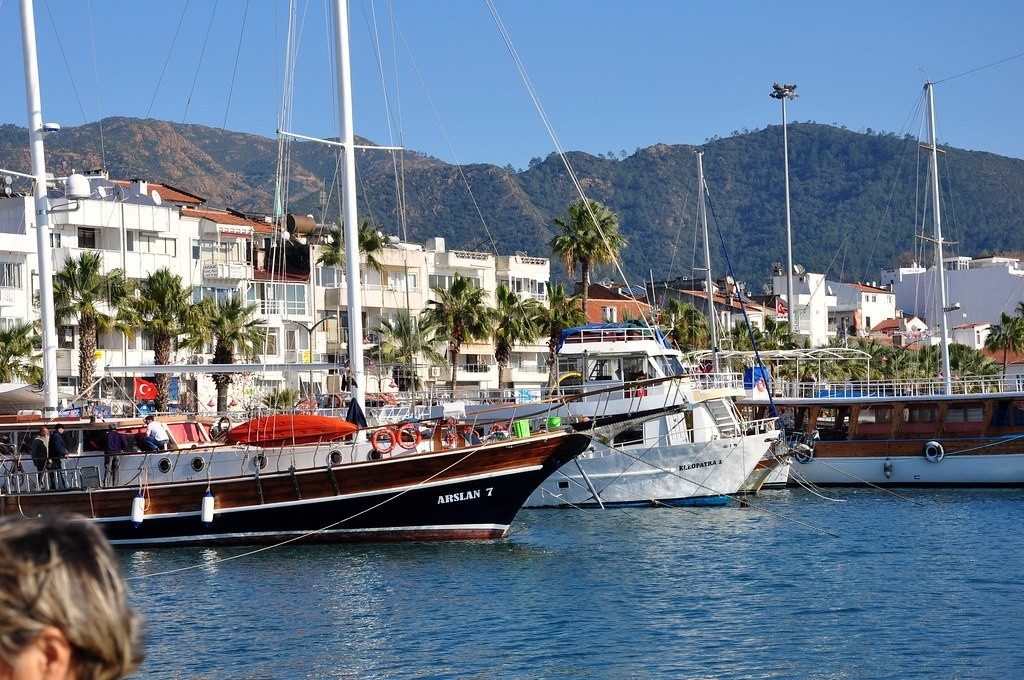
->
[548,416,562,427]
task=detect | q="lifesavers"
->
[443,433,455,444]
[488,424,504,435]
[327,394,342,408]
[218,416,231,431]
[757,377,766,392]
[372,429,397,454]
[396,423,423,449]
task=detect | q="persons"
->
[142,415,170,451]
[100,423,128,488]
[48,424,70,489]
[32,427,51,492]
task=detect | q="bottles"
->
[163,441,167,450]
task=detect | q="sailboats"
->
[0,0,1023,545]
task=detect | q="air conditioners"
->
[429,367,441,377]
[194,354,205,365]
